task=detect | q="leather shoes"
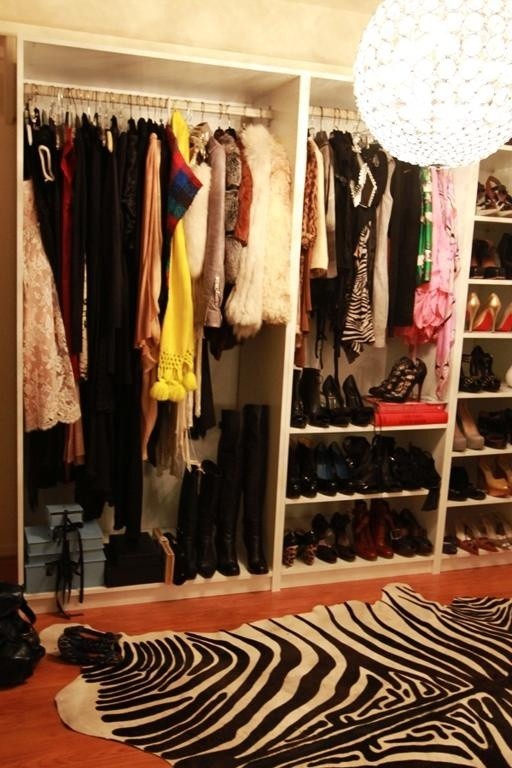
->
[287,435,442,498]
[448,455,512,501]
[282,501,434,567]
[290,367,375,429]
[468,239,506,280]
[454,399,485,451]
[477,409,511,449]
[452,511,512,557]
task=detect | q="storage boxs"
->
[360,395,448,427]
[103,532,165,587]
[24,550,107,594]
[24,525,106,564]
[42,503,84,530]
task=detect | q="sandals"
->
[459,353,481,392]
[471,346,500,392]
[474,175,512,216]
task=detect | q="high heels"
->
[368,356,427,402]
[467,292,512,331]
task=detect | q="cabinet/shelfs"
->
[270,73,482,583]
[0,21,307,617]
[433,127,511,575]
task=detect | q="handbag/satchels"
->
[0,591,46,689]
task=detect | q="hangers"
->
[307,105,378,149]
[21,83,273,153]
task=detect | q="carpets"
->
[35,581,512,768]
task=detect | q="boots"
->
[172,402,271,585]
